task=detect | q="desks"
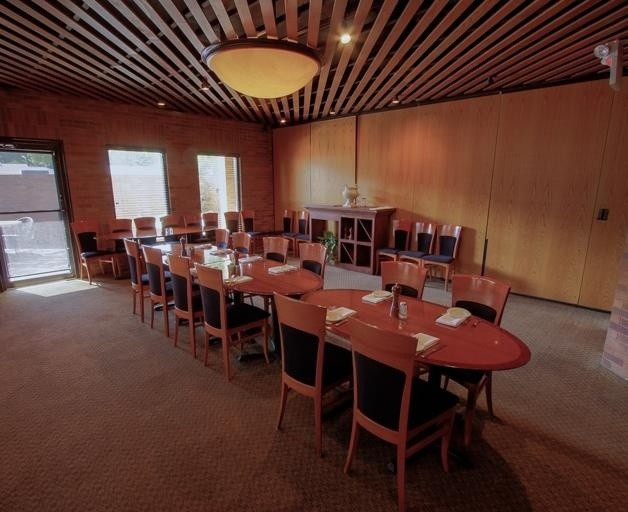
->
[0,220,30,273]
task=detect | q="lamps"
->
[199,36,328,100]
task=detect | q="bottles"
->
[389,280,401,317]
[398,301,408,319]
[179,236,187,256]
[233,249,239,265]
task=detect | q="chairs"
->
[270,290,351,458]
[340,314,464,511]
[15,216,43,261]
[417,271,513,423]
